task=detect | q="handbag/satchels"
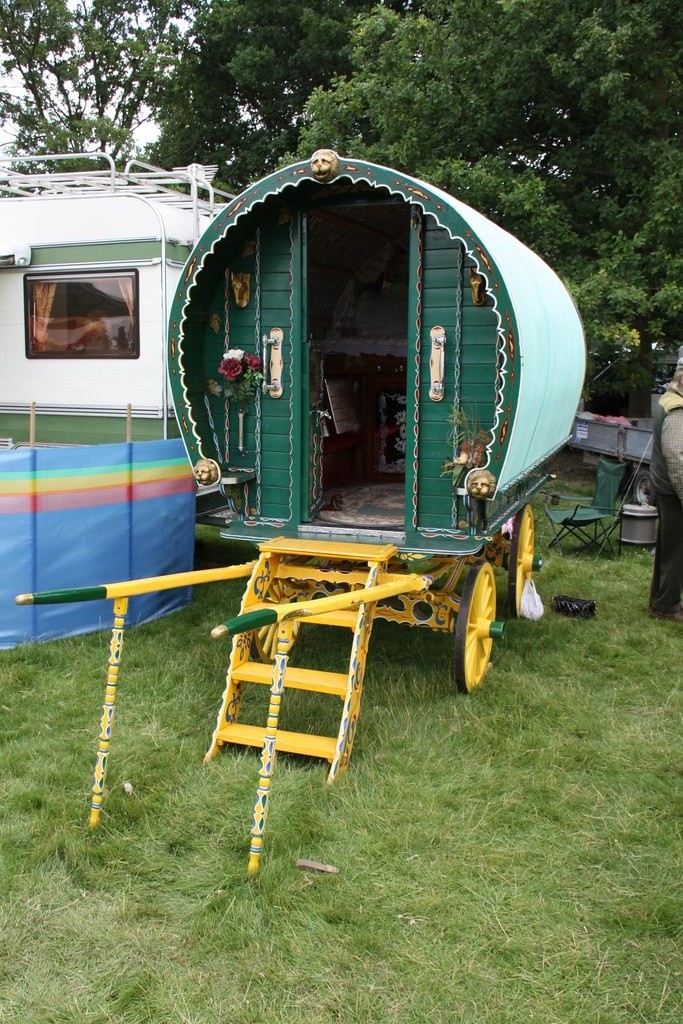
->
[520,579,544,621]
[552,595,597,617]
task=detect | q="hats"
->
[676,356,683,371]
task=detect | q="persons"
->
[649,356,683,623]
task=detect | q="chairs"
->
[538,456,626,557]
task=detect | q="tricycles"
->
[572,414,658,506]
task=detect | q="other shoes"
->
[649,607,683,621]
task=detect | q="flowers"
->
[439,395,493,487]
[208,346,267,414]
